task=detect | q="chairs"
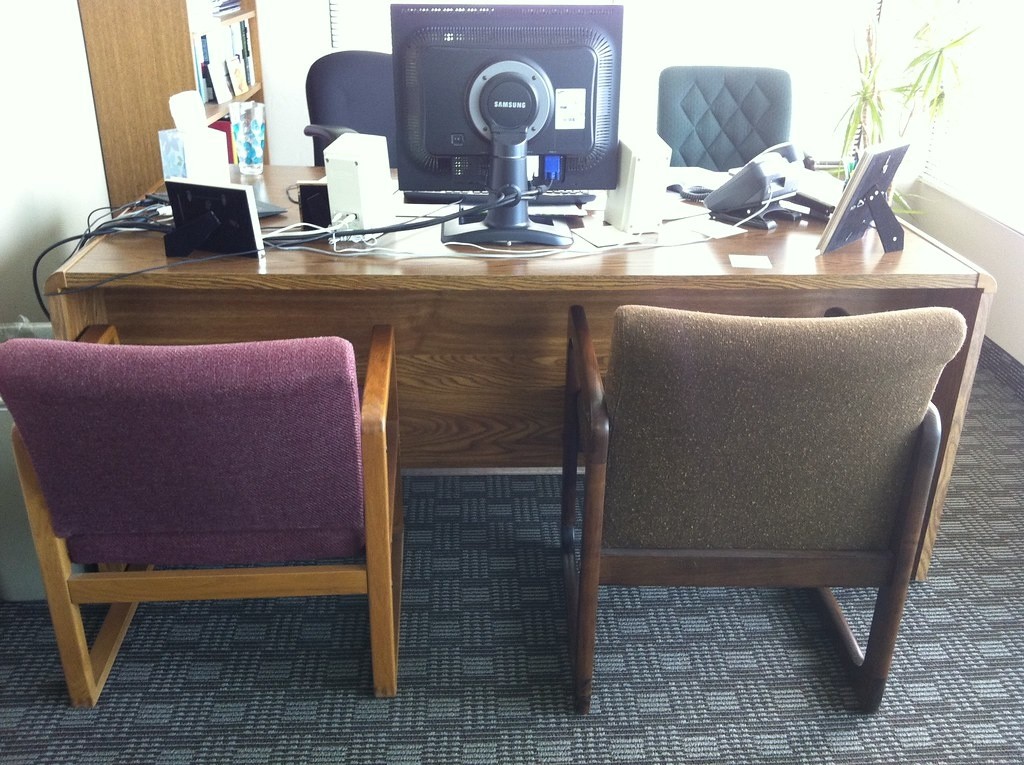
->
[550,305,971,715]
[303,47,398,169]
[656,65,791,172]
[0,325,407,709]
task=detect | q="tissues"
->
[158,90,230,190]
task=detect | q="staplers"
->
[782,189,836,223]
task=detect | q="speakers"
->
[326,134,396,242]
[603,134,672,234]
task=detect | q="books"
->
[193,0,256,106]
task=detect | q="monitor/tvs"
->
[390,4,624,246]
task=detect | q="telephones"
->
[704,140,805,231]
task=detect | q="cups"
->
[228,102,266,174]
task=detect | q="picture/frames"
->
[817,137,914,256]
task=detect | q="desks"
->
[42,166,997,580]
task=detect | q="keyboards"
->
[404,189,596,205]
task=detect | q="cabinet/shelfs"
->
[77,0,271,218]
